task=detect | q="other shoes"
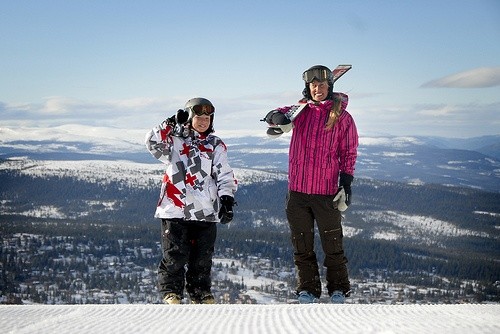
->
[296,291,314,303]
[191,298,214,304]
[163,293,182,304]
[330,292,345,303]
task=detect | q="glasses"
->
[301,68,333,83]
[190,105,215,115]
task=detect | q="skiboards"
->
[264,63,353,140]
[174,109,192,136]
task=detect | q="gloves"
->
[169,109,188,125]
[332,173,354,211]
[218,195,234,224]
[264,110,292,134]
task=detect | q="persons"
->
[260,65,359,304]
[145,98,238,304]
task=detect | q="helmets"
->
[184,98,215,125]
[303,65,333,90]
